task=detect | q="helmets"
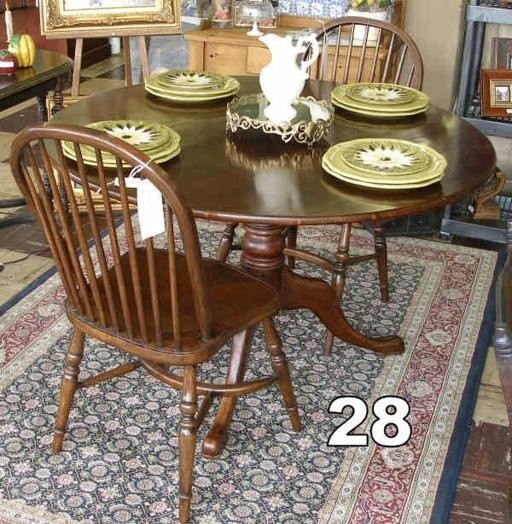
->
[144,70,242,103]
[157,164,186,190]
[60,120,182,168]
[330,82,430,118]
[143,97,240,121]
[320,136,448,189]
[317,179,444,208]
[330,113,429,134]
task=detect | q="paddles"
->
[0,209,501,524]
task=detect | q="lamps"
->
[492,215,512,431]
[217,17,423,355]
[9,122,302,522]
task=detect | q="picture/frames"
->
[0,46,74,228]
[43,73,497,461]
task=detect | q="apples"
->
[5,10,13,42]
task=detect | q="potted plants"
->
[232,0,279,28]
[480,69,512,116]
[38,0,181,40]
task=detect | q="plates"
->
[438,0,512,243]
[183,21,386,84]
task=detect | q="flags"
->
[347,9,386,41]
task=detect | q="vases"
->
[350,0,392,11]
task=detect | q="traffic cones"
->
[8,33,36,68]
